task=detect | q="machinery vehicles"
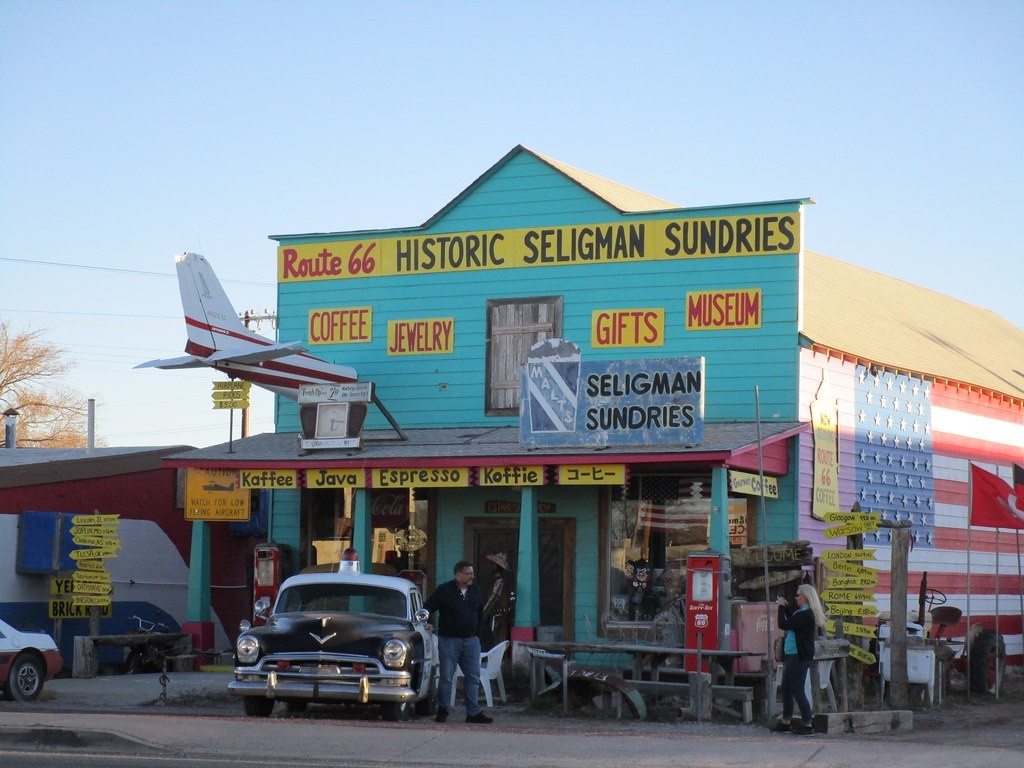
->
[907,570,1008,701]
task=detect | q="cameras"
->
[775,598,786,605]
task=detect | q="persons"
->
[482,552,511,661]
[770,583,828,735]
[423,561,494,723]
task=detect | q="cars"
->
[0,619,63,703]
[227,572,439,722]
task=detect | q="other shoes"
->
[792,725,816,736]
[770,723,791,733]
[465,710,493,724]
[436,704,449,723]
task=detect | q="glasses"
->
[795,594,799,597]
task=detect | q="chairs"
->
[770,619,934,722]
[437,638,511,706]
[304,596,337,612]
[374,598,398,617]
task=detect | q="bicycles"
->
[126,614,176,675]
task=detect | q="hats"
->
[485,554,509,571]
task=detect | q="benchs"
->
[72,632,195,679]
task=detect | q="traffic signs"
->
[818,511,882,665]
[69,514,121,606]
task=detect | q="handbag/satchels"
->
[774,637,785,662]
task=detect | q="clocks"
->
[314,402,351,439]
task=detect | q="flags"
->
[970,463,1024,534]
[640,471,709,530]
